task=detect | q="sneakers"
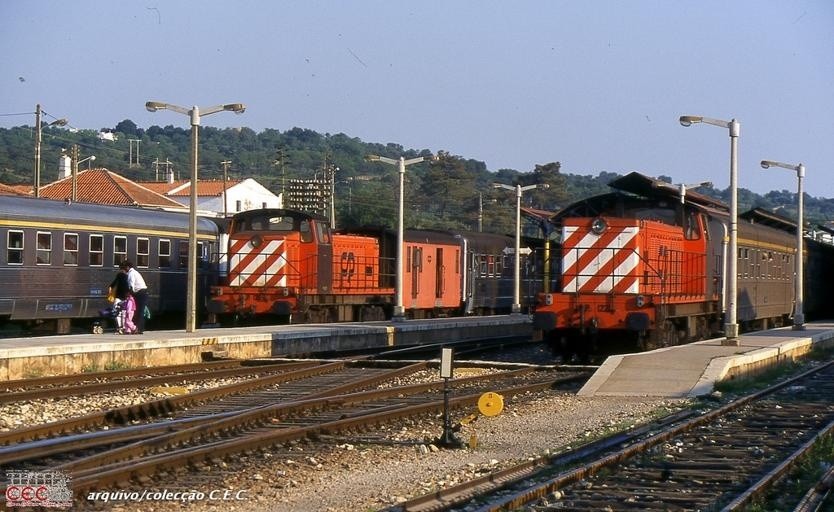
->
[114,328,145,334]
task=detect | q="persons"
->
[118,260,147,335]
[120,287,139,335]
[106,264,128,334]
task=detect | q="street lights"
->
[71,144,96,202]
[490,182,550,317]
[761,160,806,330]
[146,101,245,332]
[34,105,67,198]
[363,154,440,321]
[679,116,742,347]
